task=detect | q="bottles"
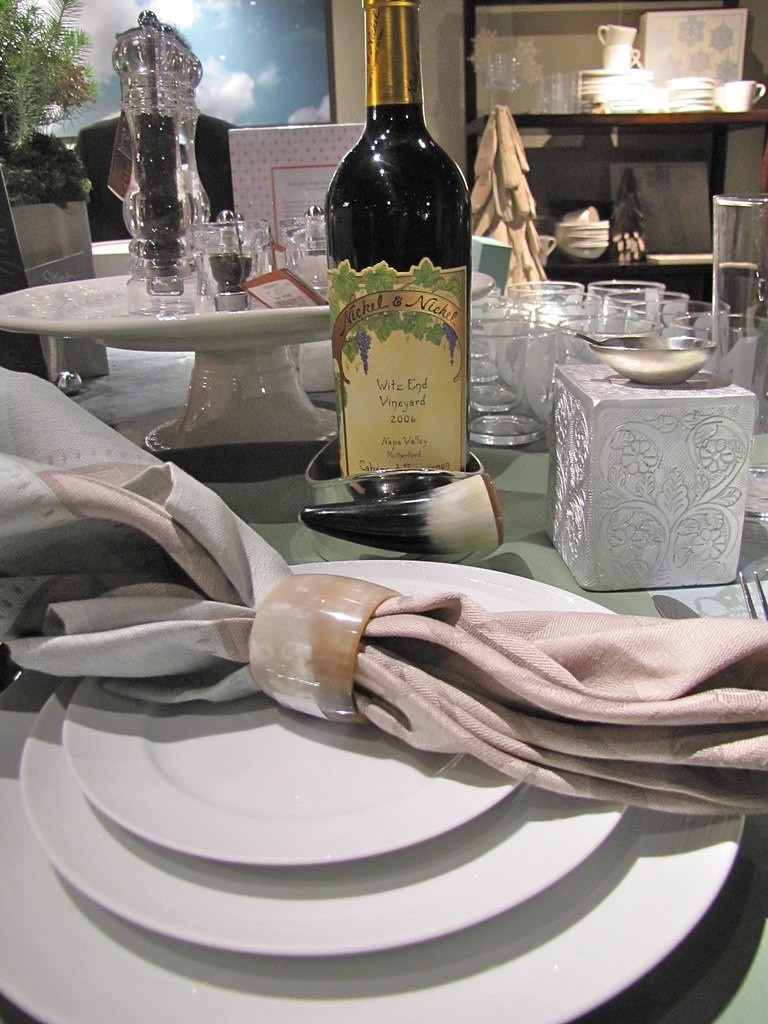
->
[322,0,471,478]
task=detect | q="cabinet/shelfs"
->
[461,1,768,298]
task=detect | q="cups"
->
[460,278,768,447]
[596,22,642,70]
[716,78,767,113]
[564,206,600,223]
[708,192,768,523]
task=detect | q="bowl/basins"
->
[588,334,718,385]
[554,220,610,260]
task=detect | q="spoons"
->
[652,593,701,619]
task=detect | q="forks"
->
[738,568,767,622]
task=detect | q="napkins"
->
[0,367,768,826]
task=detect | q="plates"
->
[61,674,524,868]
[1,557,747,1024]
[579,69,717,114]
[17,681,629,958]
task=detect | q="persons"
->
[76,23,240,243]
